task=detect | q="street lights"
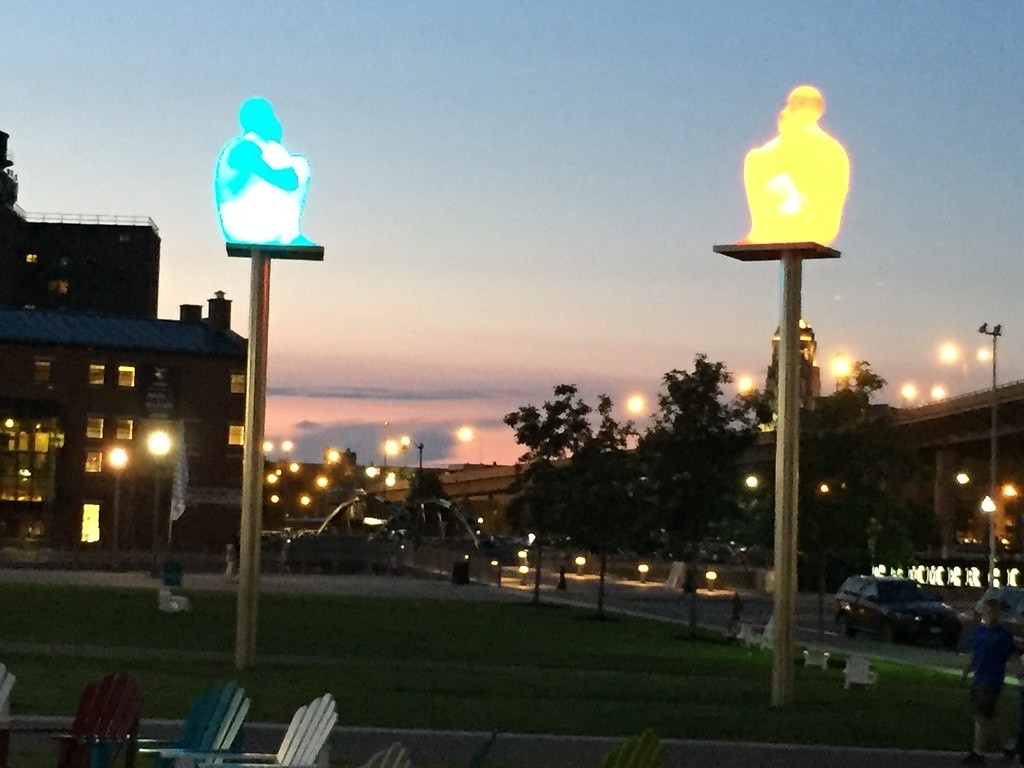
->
[979,323,1003,585]
[111,448,128,555]
[402,437,423,488]
[148,429,171,574]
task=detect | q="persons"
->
[556,566,568,591]
[728,592,742,633]
[213,97,318,246]
[225,545,239,584]
[960,599,1024,768]
[744,86,849,246]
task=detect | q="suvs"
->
[974,587,1024,639]
[831,575,963,647]
